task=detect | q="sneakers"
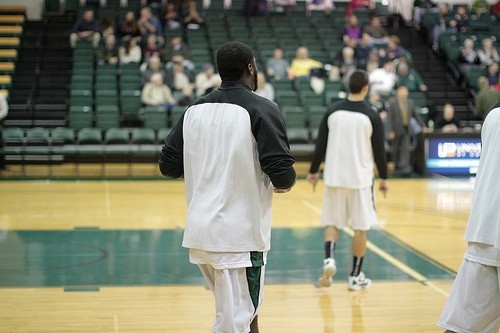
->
[349,271,372,290]
[319,259,337,286]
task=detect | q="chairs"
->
[1,0,500,181]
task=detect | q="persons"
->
[67,0,500,179]
[436,102,500,333]
[306,69,390,290]
[158,40,297,333]
[0,93,9,172]
